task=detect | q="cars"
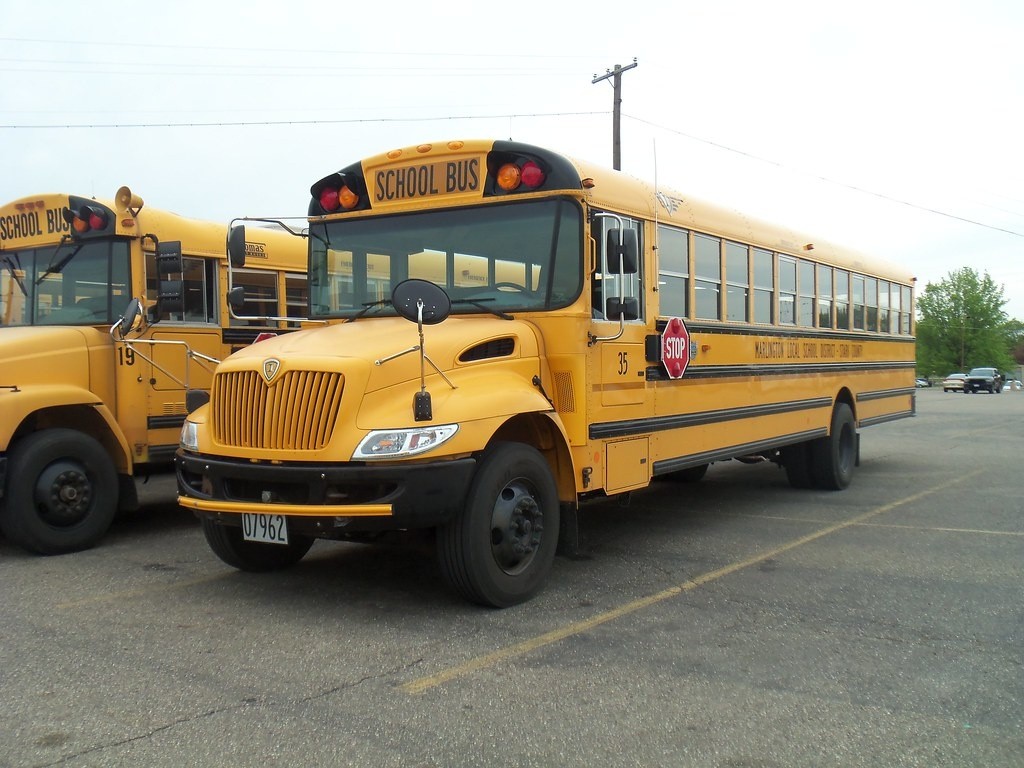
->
[915,378,929,388]
[1003,380,1023,392]
[942,373,968,392]
[964,367,1003,393]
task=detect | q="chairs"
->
[78,296,132,322]
[537,246,578,296]
[660,283,831,326]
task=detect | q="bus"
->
[1,184,540,555]
[171,141,918,612]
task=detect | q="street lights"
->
[932,371,936,388]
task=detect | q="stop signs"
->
[661,316,690,379]
